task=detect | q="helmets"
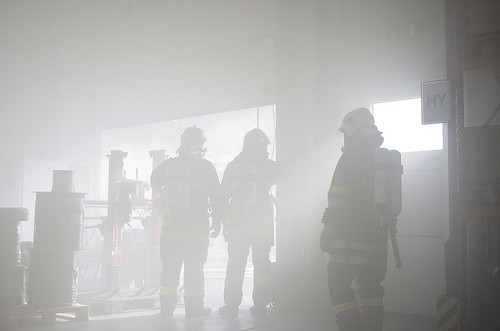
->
[181,126,206,146]
[337,107,375,137]
[244,128,270,145]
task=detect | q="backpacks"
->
[373,147,404,233]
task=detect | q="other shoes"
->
[250,307,266,319]
[160,311,173,321]
[219,304,239,319]
[186,306,212,317]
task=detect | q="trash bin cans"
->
[27,190,93,307]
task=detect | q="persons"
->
[321,107,390,330]
[152,129,219,329]
[219,129,277,320]
